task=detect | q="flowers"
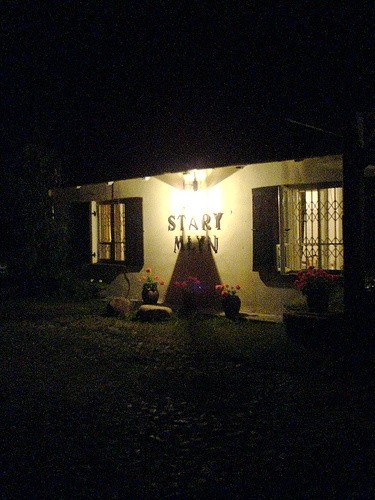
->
[137,268,165,287]
[173,276,206,292]
[294,266,342,291]
[214,282,241,298]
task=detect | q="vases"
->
[305,289,332,315]
[222,296,241,316]
[142,285,160,305]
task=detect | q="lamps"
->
[74,155,306,188]
